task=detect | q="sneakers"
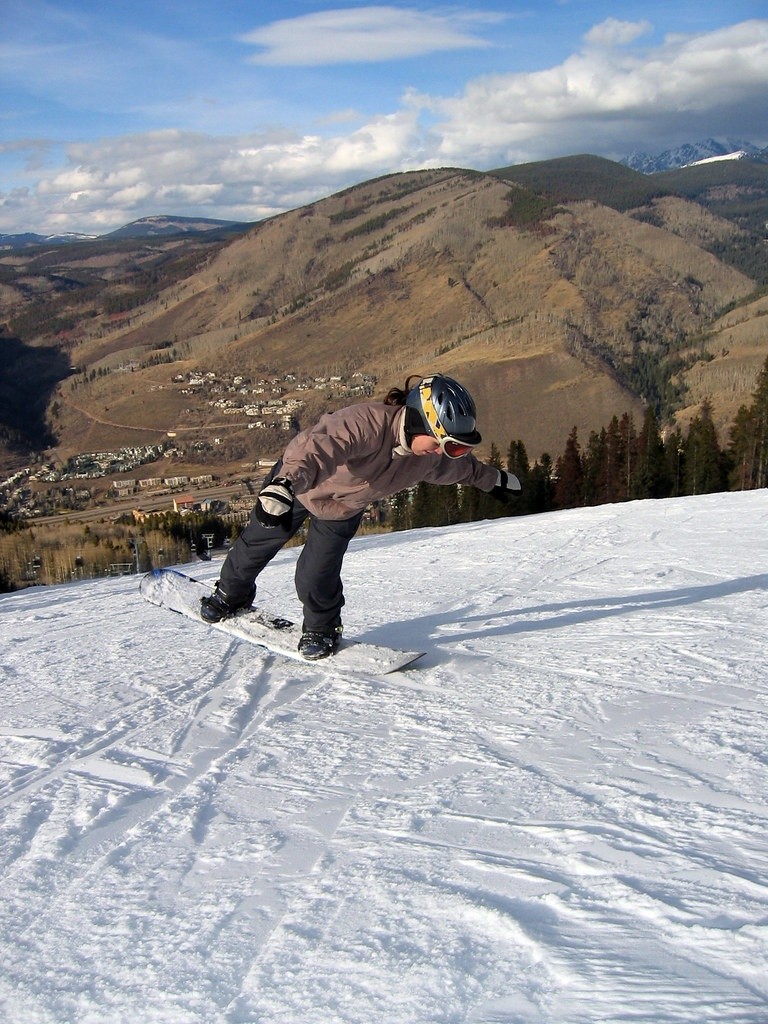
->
[298,627,342,660]
[199,581,257,623]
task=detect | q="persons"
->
[200,372,524,660]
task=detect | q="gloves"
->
[495,470,522,491]
[255,477,295,532]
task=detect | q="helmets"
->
[405,374,476,442]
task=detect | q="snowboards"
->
[136,566,430,678]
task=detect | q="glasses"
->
[419,377,481,460]
